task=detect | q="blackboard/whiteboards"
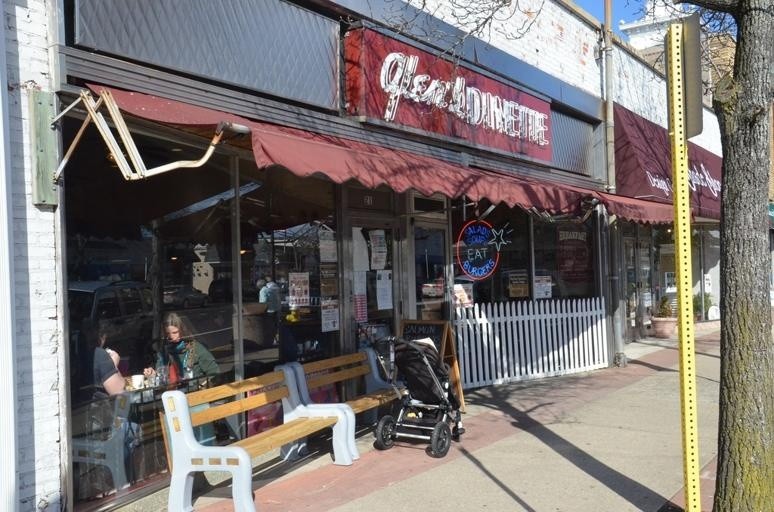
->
[399,321,448,354]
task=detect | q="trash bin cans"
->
[243,302,278,353]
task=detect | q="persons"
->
[69,316,144,500]
[143,311,221,390]
[256,278,278,325]
[265,277,281,311]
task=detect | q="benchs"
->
[71,376,216,497]
[160,347,411,512]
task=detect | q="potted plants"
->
[651,295,678,339]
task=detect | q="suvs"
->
[69,278,155,381]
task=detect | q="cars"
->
[163,284,214,311]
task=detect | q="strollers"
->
[373,335,463,460]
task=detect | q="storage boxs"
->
[506,272,553,299]
[419,283,473,321]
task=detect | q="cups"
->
[131,374,144,389]
